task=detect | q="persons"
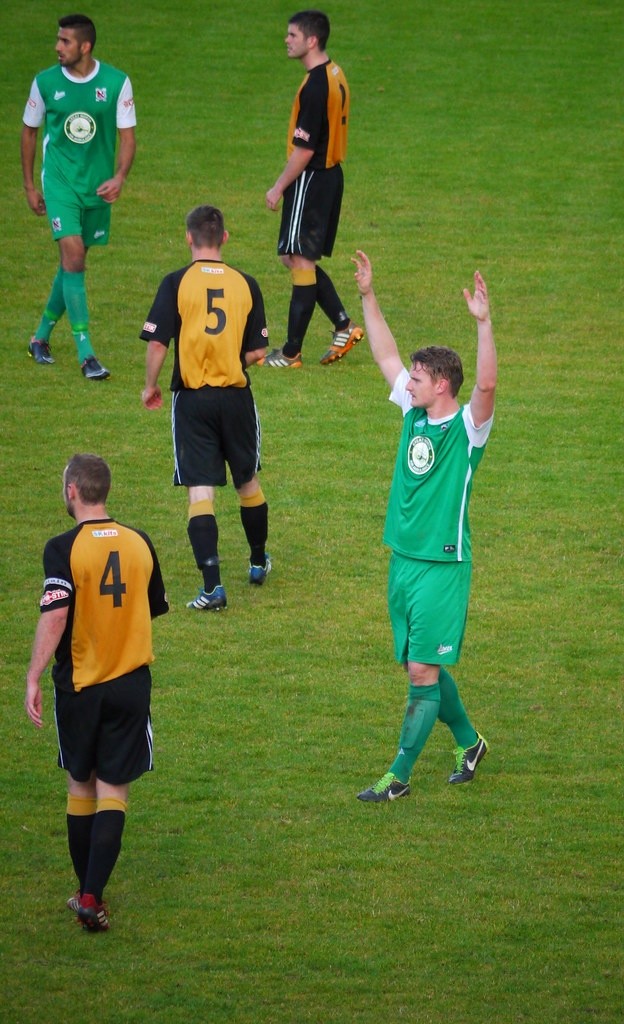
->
[24,454,170,931]
[351,250,499,801]
[21,14,137,381]
[139,206,273,611]
[256,10,365,369]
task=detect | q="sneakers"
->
[448,731,490,785]
[76,893,109,932]
[68,889,81,913]
[80,355,111,380]
[257,346,303,369]
[186,584,227,611]
[248,553,272,585]
[320,319,365,366]
[356,771,412,803]
[27,336,56,364]
